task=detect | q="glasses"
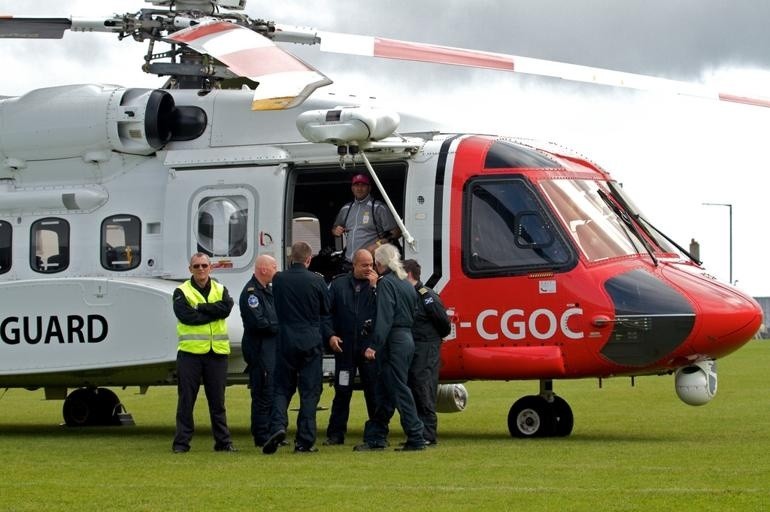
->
[194,264,208,268]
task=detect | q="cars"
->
[751,323,770,339]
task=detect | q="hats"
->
[352,175,369,185]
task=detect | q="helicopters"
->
[0,0,770,437]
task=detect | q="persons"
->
[321,248,379,445]
[263,241,333,455]
[479,220,514,262]
[239,255,290,448]
[402,259,451,445]
[352,244,426,451]
[330,173,401,273]
[173,252,238,454]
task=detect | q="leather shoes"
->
[224,445,238,451]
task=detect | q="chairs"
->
[0,246,140,273]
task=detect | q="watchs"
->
[375,239,383,246]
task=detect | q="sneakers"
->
[353,439,436,451]
[295,443,319,453]
[323,439,344,445]
[263,430,286,453]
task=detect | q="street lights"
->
[702,201,732,285]
